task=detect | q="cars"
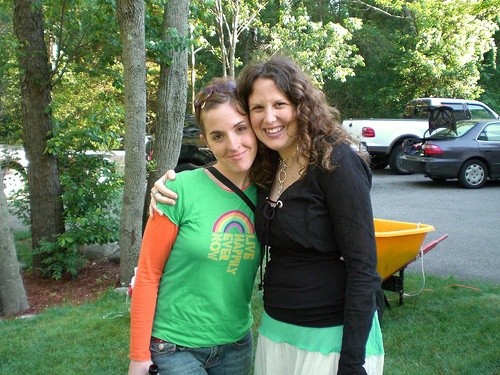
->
[398,118,500,189]
[145,115,217,172]
[0,143,29,201]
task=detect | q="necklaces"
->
[278,144,302,195]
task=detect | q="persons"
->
[149,56,392,375]
[127,77,266,375]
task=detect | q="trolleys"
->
[372,218,449,308]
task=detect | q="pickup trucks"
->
[65,135,154,186]
[342,97,500,176]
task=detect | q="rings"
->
[153,192,159,198]
